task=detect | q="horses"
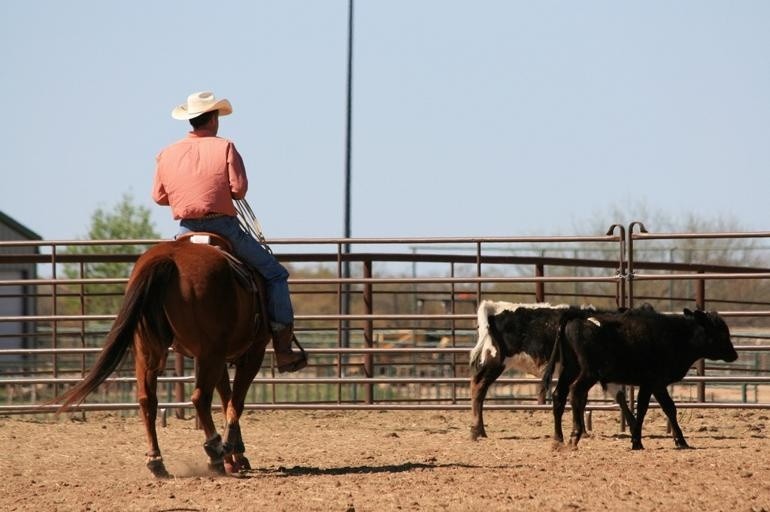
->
[44,242,271,479]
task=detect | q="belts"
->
[205,214,223,220]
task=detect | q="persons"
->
[152,92,307,372]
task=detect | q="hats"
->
[171,91,233,120]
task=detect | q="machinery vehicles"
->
[332,296,481,389]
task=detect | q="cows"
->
[469,300,656,441]
[536,308,738,451]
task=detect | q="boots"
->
[269,320,308,373]
